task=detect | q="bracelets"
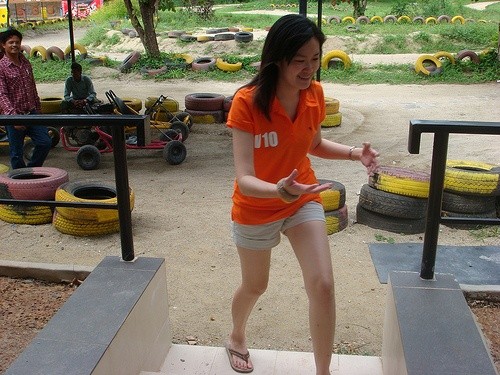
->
[349,144,358,161]
[277,178,300,203]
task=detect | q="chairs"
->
[105,90,139,129]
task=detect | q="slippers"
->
[226,344,253,373]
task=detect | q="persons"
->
[9,15,13,26]
[0,29,53,170]
[60,6,78,18]
[22,8,29,23]
[226,14,381,375]
[58,62,101,114]
